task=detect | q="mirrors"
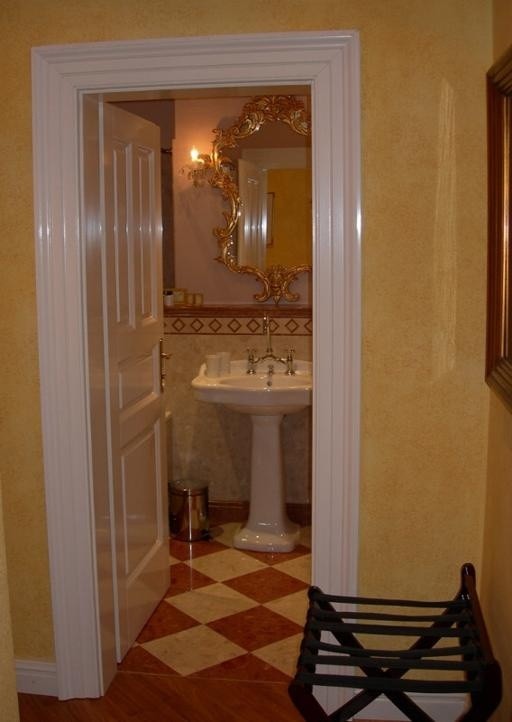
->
[203,93,311,307]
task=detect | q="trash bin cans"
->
[167,479,211,543]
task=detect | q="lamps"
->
[176,143,207,188]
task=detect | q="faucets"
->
[262,311,271,354]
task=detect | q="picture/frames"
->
[482,42,512,418]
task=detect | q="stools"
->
[269,558,503,720]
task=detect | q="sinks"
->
[191,359,313,415]
